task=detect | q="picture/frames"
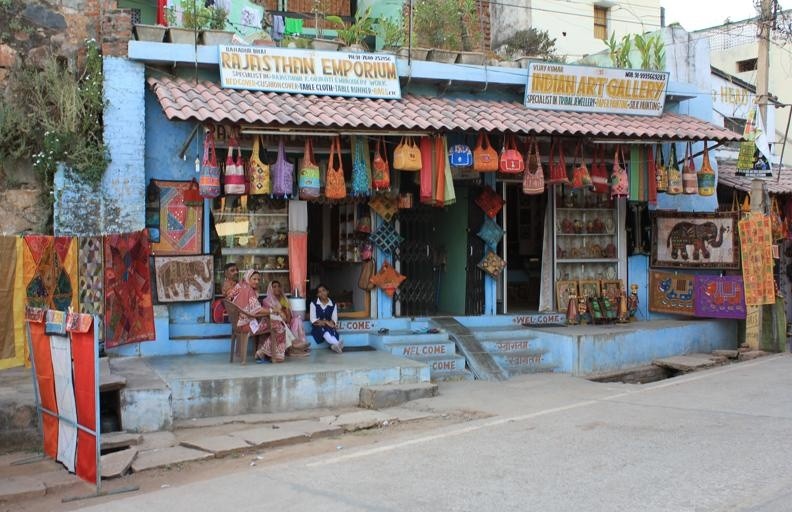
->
[557,280,623,313]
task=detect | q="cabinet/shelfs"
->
[213,212,293,298]
[553,183,619,313]
[517,185,547,255]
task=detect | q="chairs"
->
[222,299,276,366]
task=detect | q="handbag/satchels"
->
[394,133,426,172]
[297,135,321,201]
[653,144,716,197]
[198,131,221,198]
[223,127,246,197]
[248,131,271,195]
[521,131,546,195]
[270,136,296,196]
[447,131,525,174]
[546,134,631,198]
[323,135,348,201]
[351,135,392,197]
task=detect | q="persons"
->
[225,268,287,364]
[222,263,312,357]
[310,282,345,353]
[263,279,311,353]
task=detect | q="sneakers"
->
[250,339,346,361]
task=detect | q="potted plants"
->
[282,37,311,48]
[197,6,235,45]
[311,38,345,51]
[323,1,558,68]
[252,32,277,47]
[134,23,168,42]
[167,0,202,44]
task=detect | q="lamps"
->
[194,128,200,174]
[590,137,665,145]
[239,124,339,137]
[338,128,432,137]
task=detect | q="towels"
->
[242,7,304,41]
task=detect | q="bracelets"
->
[269,308,272,314]
